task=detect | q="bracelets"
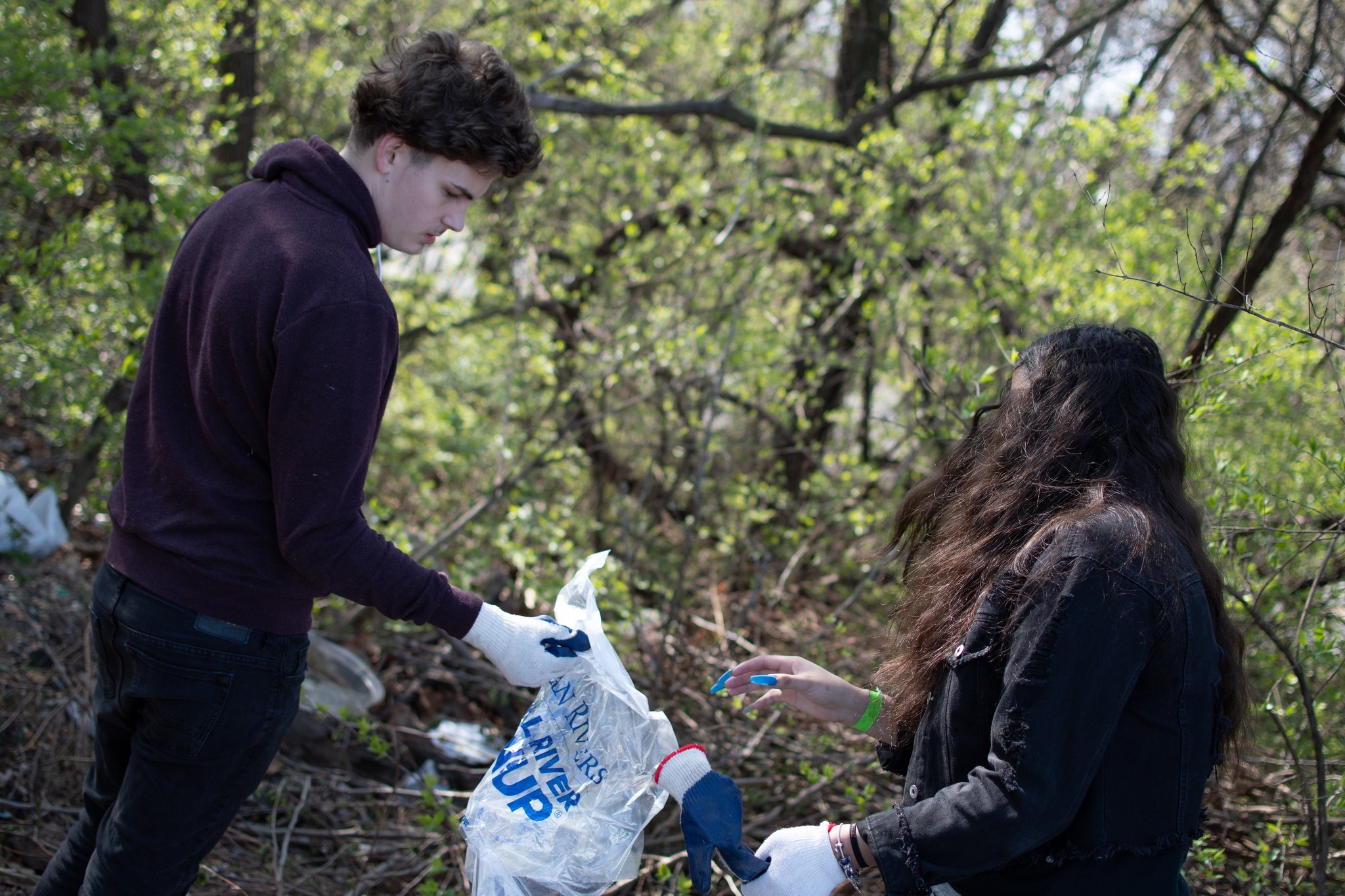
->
[850,689,881,732]
[850,823,871,868]
[834,822,863,893]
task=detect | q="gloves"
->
[738,819,867,896]
[656,743,772,896]
[464,601,590,687]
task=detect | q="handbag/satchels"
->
[461,550,680,896]
[0,471,67,558]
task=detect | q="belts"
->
[188,614,310,660]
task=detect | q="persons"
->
[32,28,594,896]
[710,322,1246,896]
